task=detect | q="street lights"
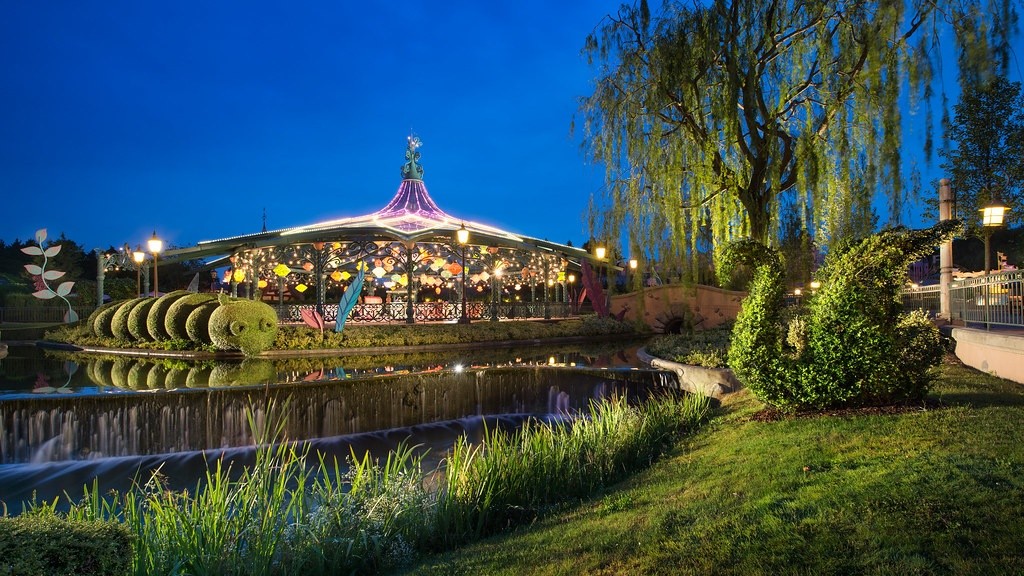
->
[133,243,146,298]
[148,230,163,298]
[938,166,1012,323]
[596,240,606,291]
[568,272,576,317]
[629,255,638,275]
[456,223,471,323]
